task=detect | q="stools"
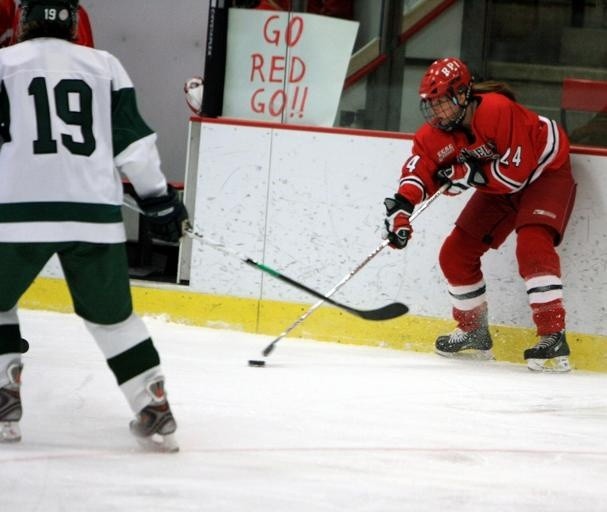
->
[118,178,185,277]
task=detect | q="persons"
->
[384,57,577,360]
[0,1,189,436]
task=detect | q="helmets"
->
[418,57,474,99]
[18,0,80,41]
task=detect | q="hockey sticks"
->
[262,180,452,357]
[125,200,409,320]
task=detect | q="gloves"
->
[433,162,489,196]
[126,182,190,247]
[381,192,416,250]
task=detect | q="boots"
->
[523,328,571,360]
[435,301,494,351]
[128,376,177,437]
[0,360,24,422]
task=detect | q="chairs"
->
[559,78,607,152]
